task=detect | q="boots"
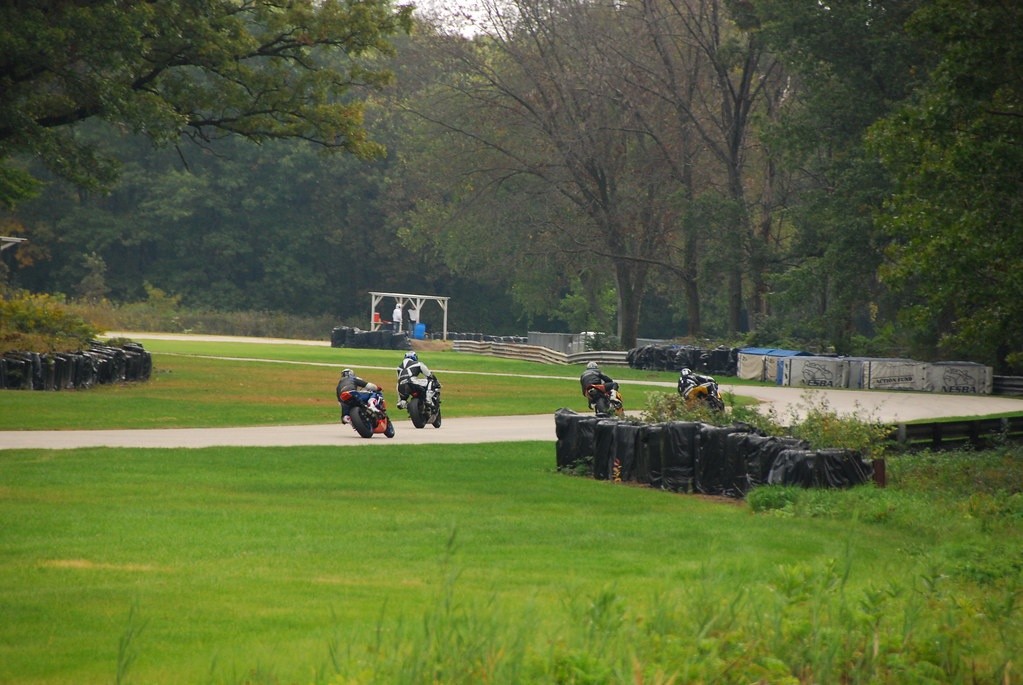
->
[426,390,436,409]
[343,415,357,431]
[609,389,621,405]
[589,403,596,411]
[368,398,381,414]
[396,400,409,409]
[711,391,718,403]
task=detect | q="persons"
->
[392,303,401,330]
[581,362,620,410]
[396,351,439,410]
[408,305,416,324]
[336,369,383,426]
[678,368,718,403]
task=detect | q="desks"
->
[370,321,403,332]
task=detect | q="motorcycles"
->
[407,378,442,428]
[339,387,395,438]
[685,382,724,413]
[585,382,624,419]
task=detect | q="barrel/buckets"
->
[413,322,426,340]
[374,313,379,322]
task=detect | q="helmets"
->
[587,362,598,370]
[681,368,692,376]
[405,352,418,361]
[341,369,354,377]
